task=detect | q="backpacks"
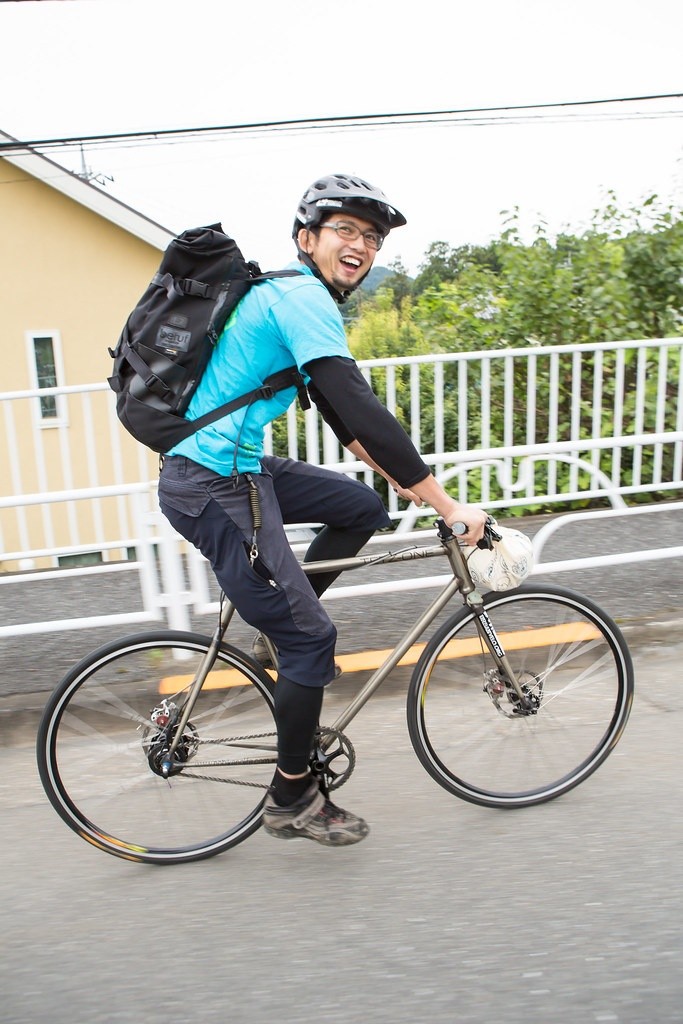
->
[106,222,311,454]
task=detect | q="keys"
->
[250,549,258,567]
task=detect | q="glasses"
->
[320,219,384,251]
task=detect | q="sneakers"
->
[263,782,369,848]
[251,630,343,679]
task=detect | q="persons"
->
[159,173,489,847]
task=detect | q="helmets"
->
[292,174,407,239]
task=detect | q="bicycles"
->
[34,486,637,867]
